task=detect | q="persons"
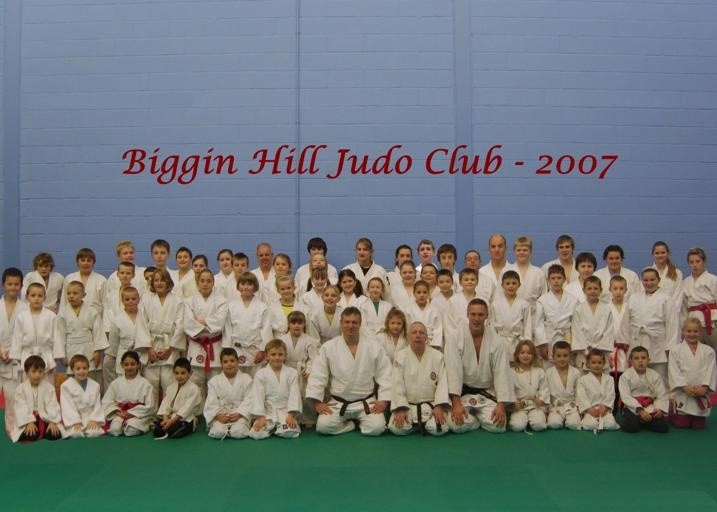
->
[223,252,249,302]
[169,247,193,297]
[59,354,106,439]
[682,248,717,353]
[618,345,671,433]
[305,307,391,436]
[436,243,460,283]
[497,236,548,335]
[249,339,303,440]
[250,243,275,292]
[100,351,156,437]
[101,262,135,338]
[311,285,344,345]
[444,298,516,434]
[509,339,551,434]
[387,321,452,436]
[404,281,444,349]
[153,239,173,272]
[390,261,417,309]
[143,267,157,289]
[221,271,269,380]
[475,233,516,305]
[668,318,717,429]
[53,280,111,396]
[430,270,456,308]
[181,255,208,296]
[56,248,107,317]
[270,274,308,341]
[0,268,24,440]
[615,266,676,386]
[133,266,184,411]
[420,264,437,297]
[278,311,318,428]
[359,276,391,335]
[213,249,235,298]
[261,253,291,304]
[606,275,629,373]
[377,307,407,360]
[570,276,614,375]
[575,349,620,434]
[415,239,438,272]
[7,283,57,394]
[341,238,386,298]
[299,264,330,316]
[541,235,579,291]
[203,348,253,441]
[465,250,481,270]
[184,268,229,398]
[297,253,328,297]
[564,252,612,307]
[20,252,64,313]
[294,238,338,287]
[592,245,639,306]
[546,340,581,431]
[105,241,147,296]
[154,358,202,441]
[534,264,579,370]
[104,286,141,380]
[336,269,366,308]
[491,271,532,363]
[391,244,412,272]
[10,355,62,443]
[445,267,491,344]
[640,241,682,296]
